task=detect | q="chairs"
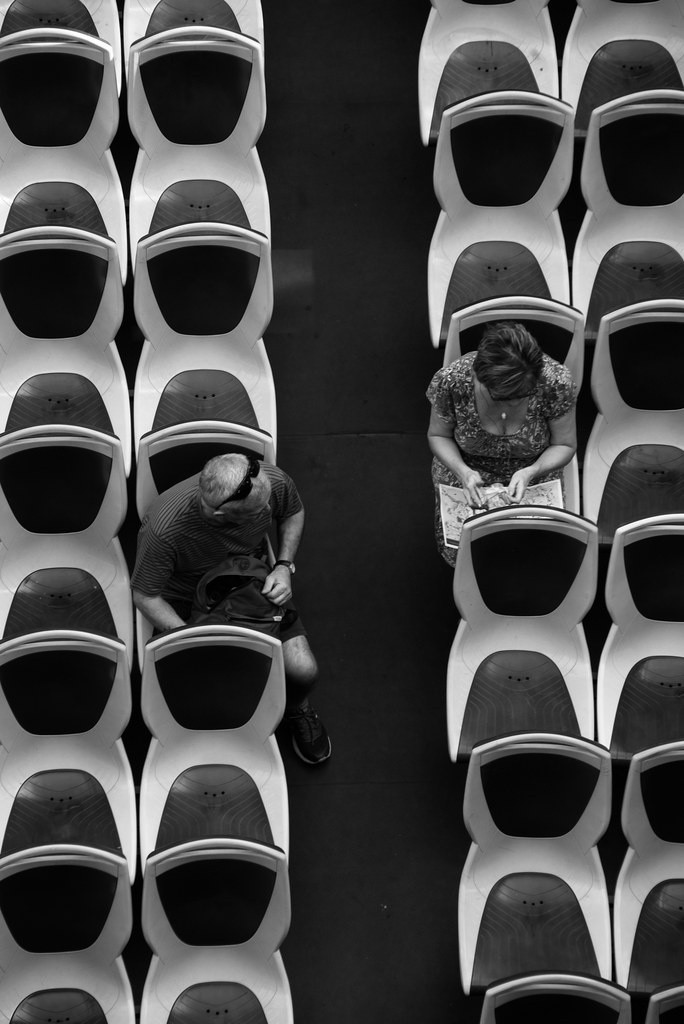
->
[0,0,296,1024]
[417,0,684,1024]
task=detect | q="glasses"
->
[485,381,538,401]
[215,456,260,511]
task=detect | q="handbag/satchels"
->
[188,556,287,639]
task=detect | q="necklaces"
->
[501,413,506,419]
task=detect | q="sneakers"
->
[284,704,331,764]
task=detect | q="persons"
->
[130,453,331,764]
[425,322,577,569]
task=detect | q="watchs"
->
[273,560,295,575]
